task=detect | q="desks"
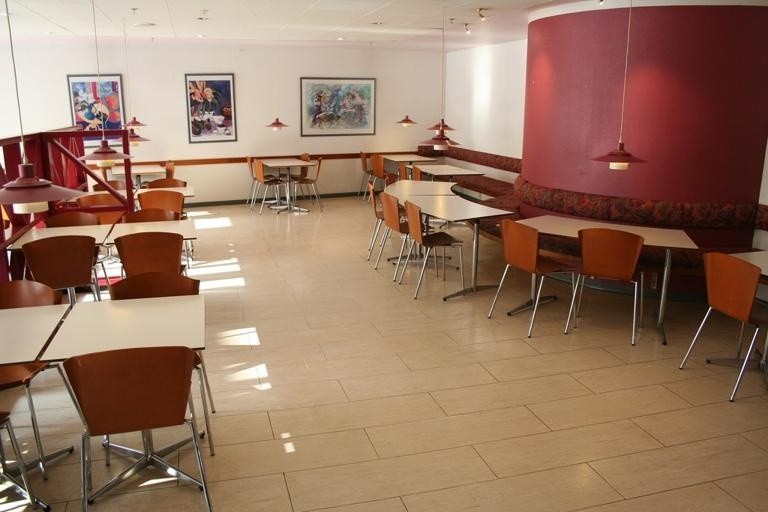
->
[705,250,768,386]
[380,153,486,184]
[513,213,699,349]
[1,301,75,484]
[40,292,215,505]
[383,177,515,305]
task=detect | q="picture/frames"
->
[300,76,376,137]
[184,74,237,144]
[67,75,127,148]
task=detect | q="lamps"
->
[589,0,649,171]
[464,23,471,35]
[394,7,462,152]
[77,0,150,168]
[477,8,485,21]
[0,0,75,214]
[265,118,288,131]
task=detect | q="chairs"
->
[373,191,435,283]
[486,217,578,338]
[677,251,768,401]
[397,200,468,301]
[106,270,219,413]
[0,407,38,509]
[246,154,322,215]
[357,151,422,206]
[0,278,91,478]
[365,179,406,262]
[1,159,200,300]
[563,226,646,347]
[61,344,217,510]
